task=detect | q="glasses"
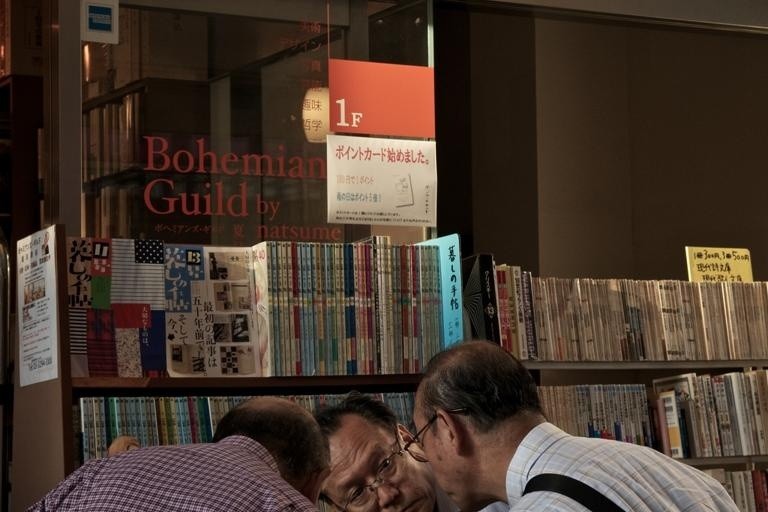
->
[403,407,469,463]
[335,427,404,512]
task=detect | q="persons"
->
[26,394,330,511]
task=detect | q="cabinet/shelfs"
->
[1,74,212,313]
[9,223,768,511]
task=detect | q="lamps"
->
[302,87,330,144]
